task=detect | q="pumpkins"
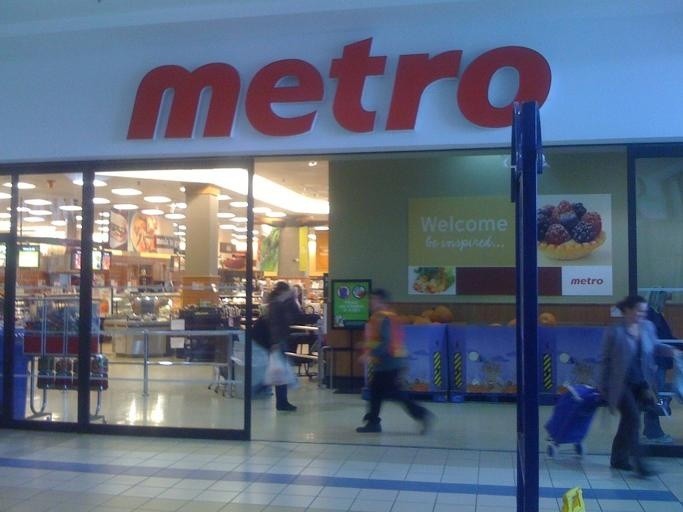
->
[397,305,454,326]
[538,312,555,325]
[509,319,516,325]
[409,377,570,394]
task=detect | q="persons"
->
[595,294,677,476]
[356,288,438,436]
[252,277,257,292]
[643,286,683,443]
[294,285,302,311]
[262,281,304,410]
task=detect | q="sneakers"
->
[420,413,437,435]
[357,417,381,432]
[610,458,660,475]
[276,402,297,411]
[640,432,672,444]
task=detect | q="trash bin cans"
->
[0,328,33,420]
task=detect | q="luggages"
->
[544,384,602,456]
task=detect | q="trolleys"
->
[208,336,236,397]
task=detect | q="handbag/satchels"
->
[252,316,281,347]
[653,397,672,417]
